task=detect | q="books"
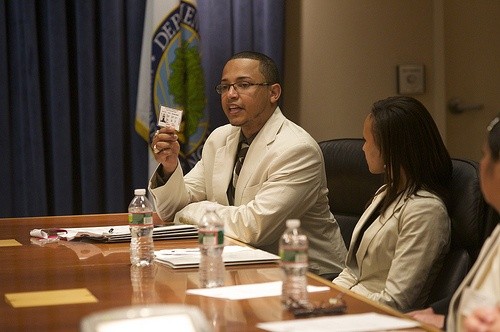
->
[152,245,283,270]
[58,225,200,242]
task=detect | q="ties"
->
[227,139,250,208]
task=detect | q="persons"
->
[409,297,448,330]
[446,117,500,332]
[148,51,348,273]
[332,96,462,314]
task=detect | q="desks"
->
[0,213,446,332]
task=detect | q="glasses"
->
[288,293,347,317]
[216,80,274,94]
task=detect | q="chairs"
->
[317,138,483,309]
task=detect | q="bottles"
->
[128,189,154,267]
[279,219,309,307]
[198,206,225,288]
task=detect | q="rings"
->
[153,145,160,154]
